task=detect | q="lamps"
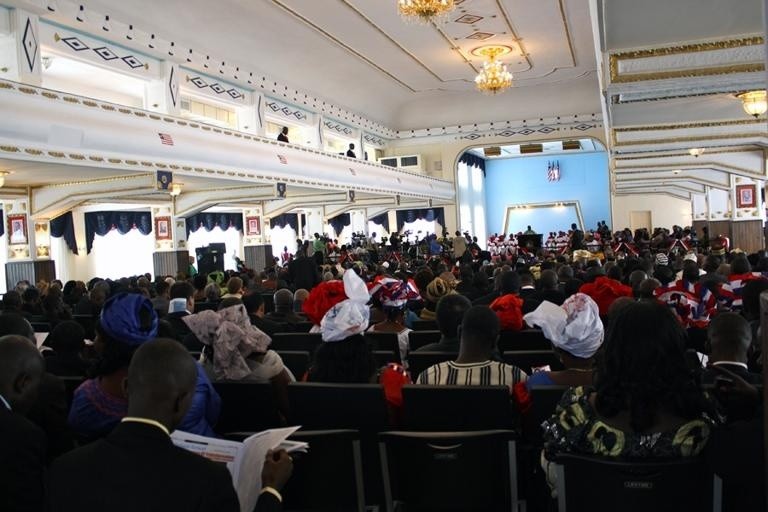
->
[393,0,457,28]
[471,42,514,98]
[735,87,767,120]
[687,147,707,159]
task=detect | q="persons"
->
[347,143,356,158]
[1,220,768,512]
[277,126,288,142]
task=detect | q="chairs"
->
[0,230,767,512]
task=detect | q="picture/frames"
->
[5,211,29,246]
[153,216,171,241]
[244,215,262,238]
[734,183,757,210]
